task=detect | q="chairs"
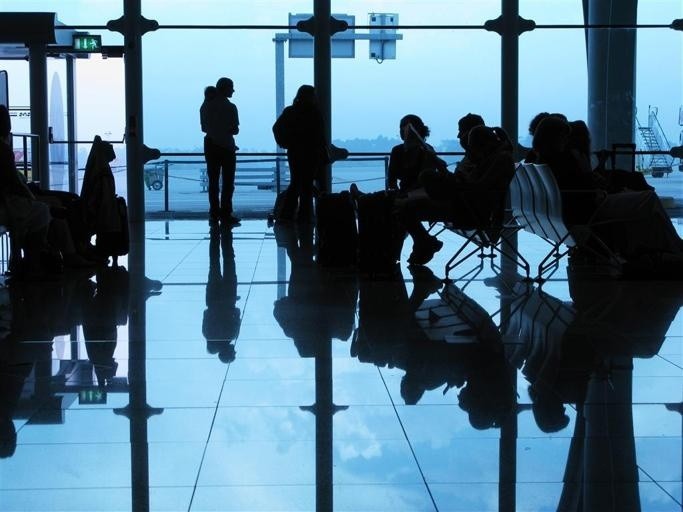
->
[417,163,578,284]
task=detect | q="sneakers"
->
[208,211,241,224]
[409,234,443,264]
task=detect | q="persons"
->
[201,221,243,364]
[199,85,224,221]
[206,76,243,228]
[202,272,220,355]
[393,125,519,269]
[525,110,659,199]
[271,228,322,359]
[530,112,683,262]
[0,281,58,460]
[348,111,488,221]
[356,268,469,408]
[383,113,445,190]
[0,103,54,281]
[271,83,336,223]
